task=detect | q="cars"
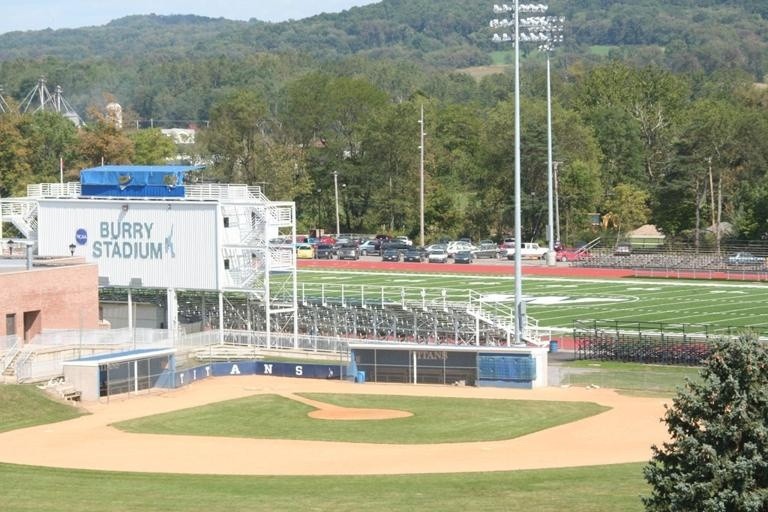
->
[339,245,360,260]
[403,250,421,262]
[428,250,449,263]
[382,249,401,262]
[575,241,589,251]
[615,246,631,257]
[553,240,563,251]
[455,251,474,264]
[723,253,765,266]
[270,234,514,260]
[556,246,593,262]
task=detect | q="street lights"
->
[704,157,716,233]
[317,187,321,238]
[331,171,340,238]
[488,0,565,346]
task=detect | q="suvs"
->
[502,242,549,260]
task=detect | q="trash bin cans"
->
[357,371,365,382]
[550,341,558,352]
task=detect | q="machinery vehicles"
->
[587,212,617,232]
